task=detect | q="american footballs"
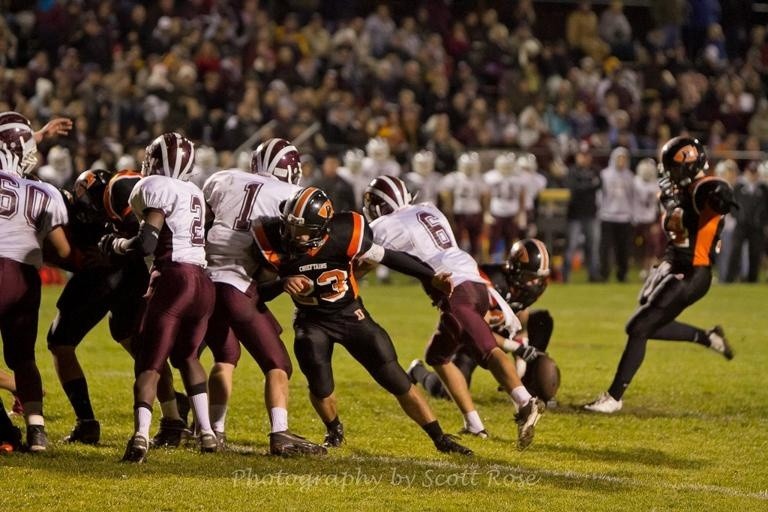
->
[527,355,563,402]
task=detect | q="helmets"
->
[660,134,706,188]
[58,131,195,225]
[250,137,334,247]
[503,238,549,305]
[363,175,412,224]
[0,111,38,179]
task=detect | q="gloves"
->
[97,233,125,263]
[517,344,549,363]
[709,184,741,216]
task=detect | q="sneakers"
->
[270,423,344,458]
[1,421,100,453]
[122,391,227,463]
[709,325,733,359]
[406,359,425,385]
[434,396,545,457]
[583,392,623,413]
